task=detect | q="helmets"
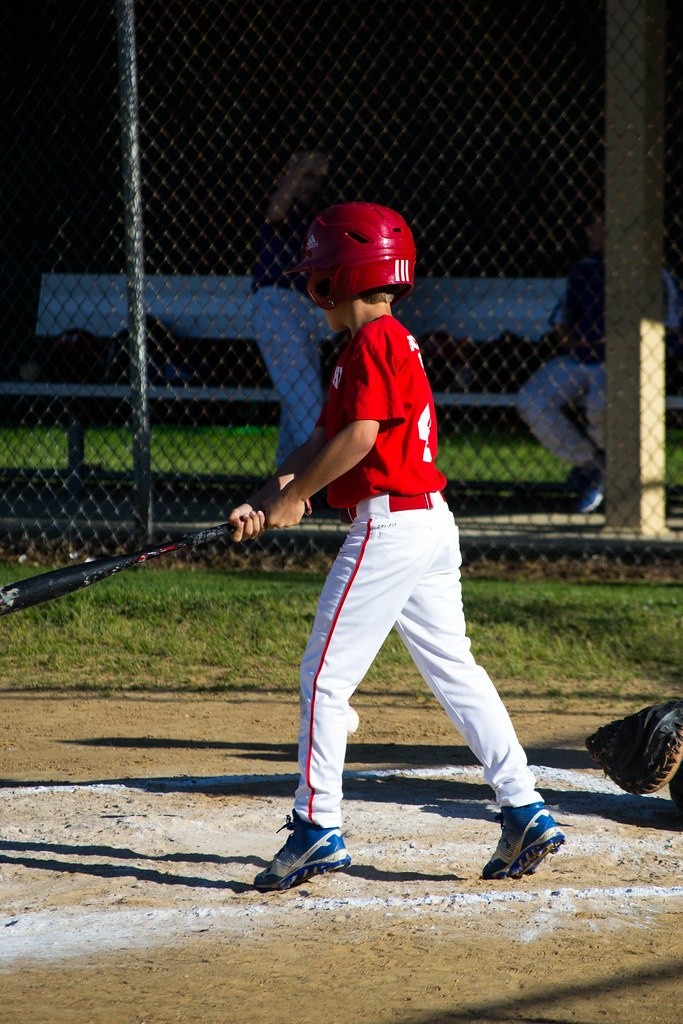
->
[281,202,417,308]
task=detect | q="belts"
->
[347,491,448,523]
[252,279,289,288]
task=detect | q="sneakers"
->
[253,810,350,890]
[482,802,563,880]
[580,477,608,514]
[565,450,606,487]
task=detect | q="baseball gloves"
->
[584,701,683,795]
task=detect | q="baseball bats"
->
[0,495,311,616]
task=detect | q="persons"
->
[230,204,567,892]
[250,137,345,469]
[514,214,678,515]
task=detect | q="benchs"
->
[0,270,682,470]
